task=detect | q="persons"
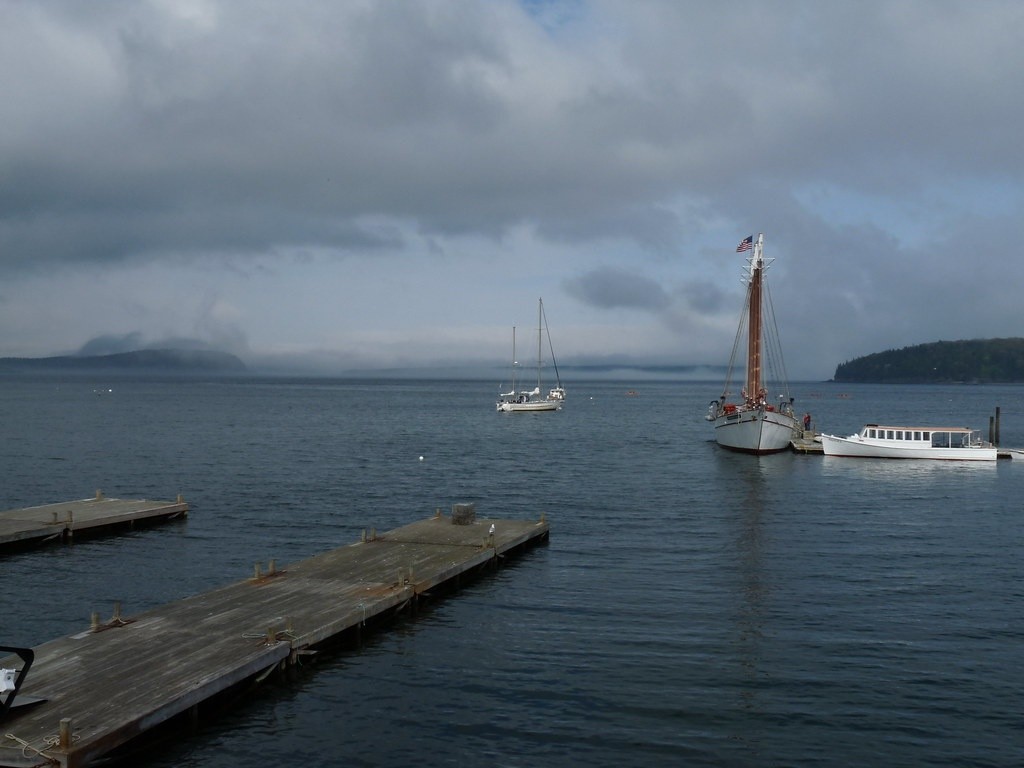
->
[803,412,810,431]
[489,524,495,542]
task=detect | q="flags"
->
[736,236,752,252]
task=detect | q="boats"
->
[820,422,998,462]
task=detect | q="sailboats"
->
[704,232,803,456]
[495,296,566,414]
